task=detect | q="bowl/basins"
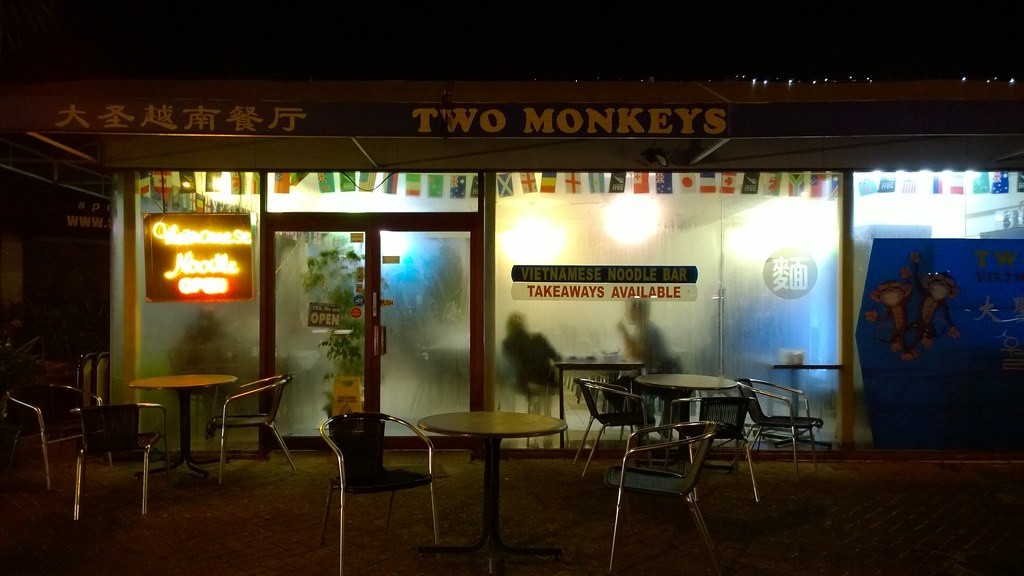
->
[606,355,623,363]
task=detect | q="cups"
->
[782,351,803,364]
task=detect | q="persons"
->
[618,297,672,375]
[501,311,565,390]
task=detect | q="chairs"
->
[5,382,174,520]
[204,374,296,484]
[573,378,824,576]
[320,413,440,576]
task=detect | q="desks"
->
[68,352,109,471]
[552,355,646,449]
[129,374,238,479]
[635,374,737,474]
[771,364,844,450]
[417,411,568,576]
